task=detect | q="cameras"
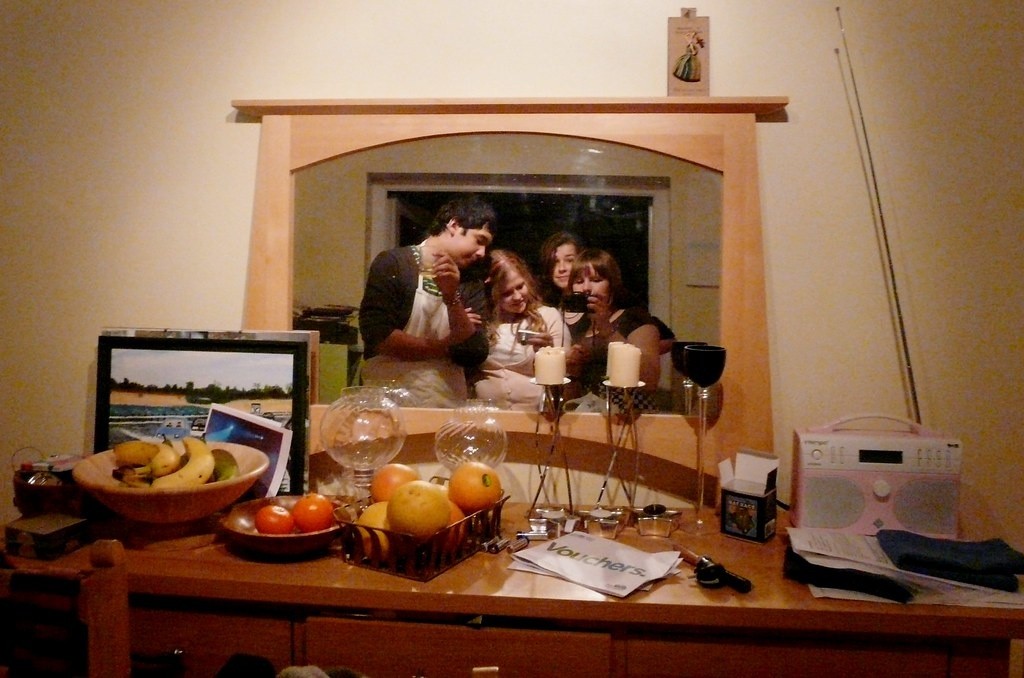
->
[518,330,540,346]
[562,289,594,313]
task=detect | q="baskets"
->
[332,476,511,583]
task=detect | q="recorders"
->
[790,426,962,541]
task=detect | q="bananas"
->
[111,437,240,489]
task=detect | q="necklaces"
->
[557,308,578,319]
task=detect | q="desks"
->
[0,495,1023,678]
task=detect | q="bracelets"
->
[444,290,461,306]
[598,327,616,341]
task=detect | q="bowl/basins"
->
[74,441,271,539]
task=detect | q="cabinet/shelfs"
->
[318,343,366,404]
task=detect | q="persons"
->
[477,233,678,415]
[358,197,497,406]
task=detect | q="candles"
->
[535,352,565,384]
[610,345,640,387]
[607,342,627,376]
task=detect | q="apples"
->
[254,491,334,535]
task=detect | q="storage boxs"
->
[718,449,781,544]
[5,512,96,560]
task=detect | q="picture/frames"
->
[95,332,308,498]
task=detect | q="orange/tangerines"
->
[358,461,501,564]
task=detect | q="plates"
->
[219,495,354,555]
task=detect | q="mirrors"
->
[231,96,789,514]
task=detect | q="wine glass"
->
[678,345,726,533]
[671,341,707,415]
